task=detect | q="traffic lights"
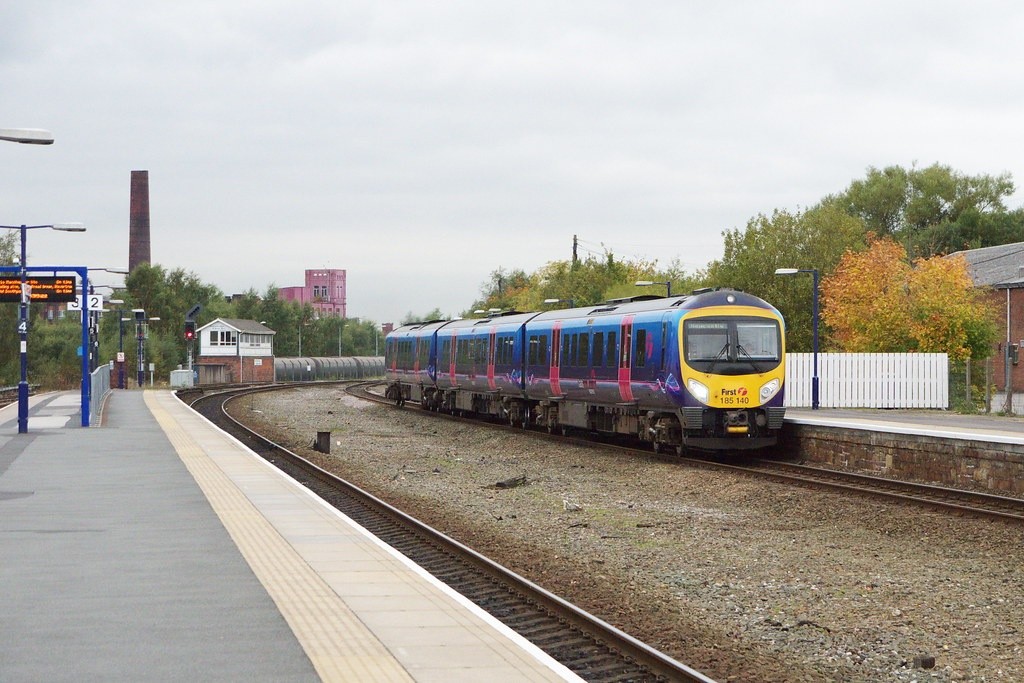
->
[185,320,196,341]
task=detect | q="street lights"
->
[121,317,161,388]
[635,280,670,297]
[97,308,123,391]
[299,323,309,358]
[774,266,821,410]
[338,325,350,357]
[77,265,130,428]
[544,298,573,309]
[94,298,124,368]
[1,223,85,435]
[88,283,126,403]
[375,323,383,356]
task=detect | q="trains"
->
[381,286,785,463]
